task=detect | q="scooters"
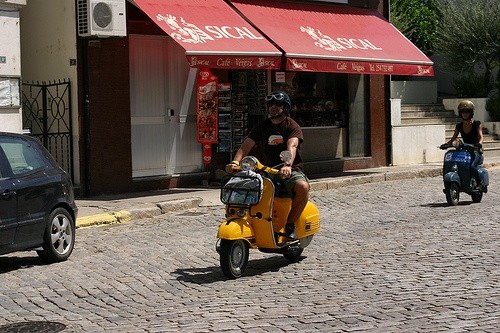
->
[440,138,489,206]
[215,151,320,279]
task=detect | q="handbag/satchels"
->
[222,171,263,205]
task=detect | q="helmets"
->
[458,101,475,111]
[266,90,290,116]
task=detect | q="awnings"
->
[133,0,434,76]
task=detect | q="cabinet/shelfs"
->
[211,73,343,162]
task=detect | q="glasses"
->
[267,101,283,107]
[461,110,470,114]
[265,92,284,102]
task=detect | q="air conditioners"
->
[77,0,127,37]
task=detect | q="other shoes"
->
[285,222,296,240]
[472,183,484,192]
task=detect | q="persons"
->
[225,91,310,240]
[441,100,484,194]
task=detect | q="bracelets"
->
[232,161,239,166]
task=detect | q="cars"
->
[0,132,79,262]
